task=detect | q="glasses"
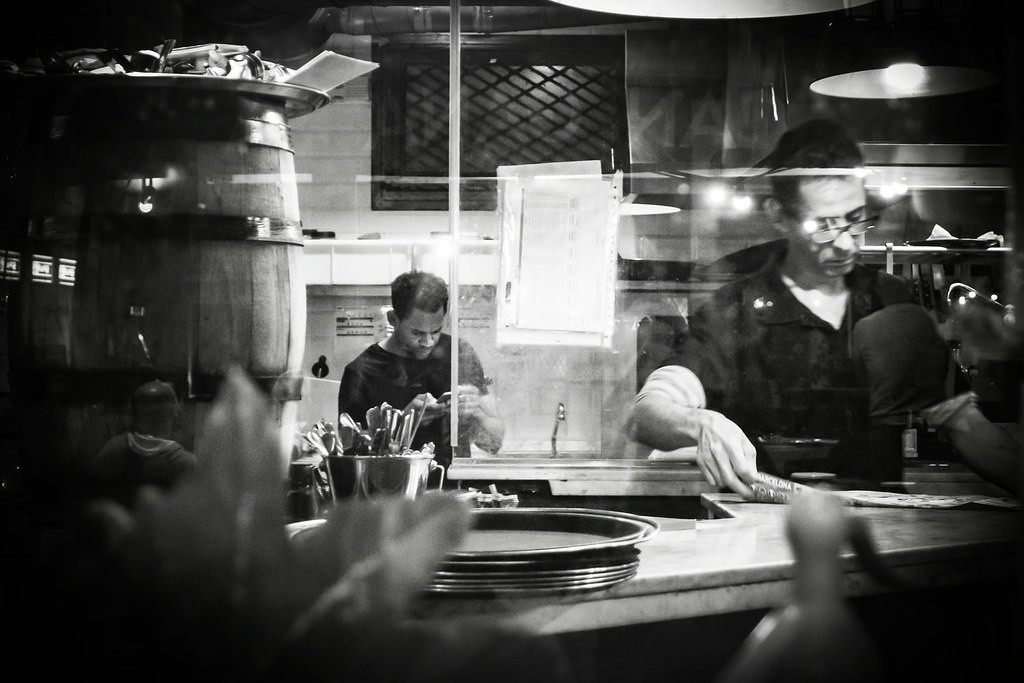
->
[782,201,882,246]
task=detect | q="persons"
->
[98,382,198,491]
[338,272,504,489]
[624,118,1024,501]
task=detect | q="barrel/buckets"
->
[29,85,309,529]
[326,454,437,505]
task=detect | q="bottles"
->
[715,490,893,683]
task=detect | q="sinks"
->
[445,447,709,480]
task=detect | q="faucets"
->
[551,402,565,457]
